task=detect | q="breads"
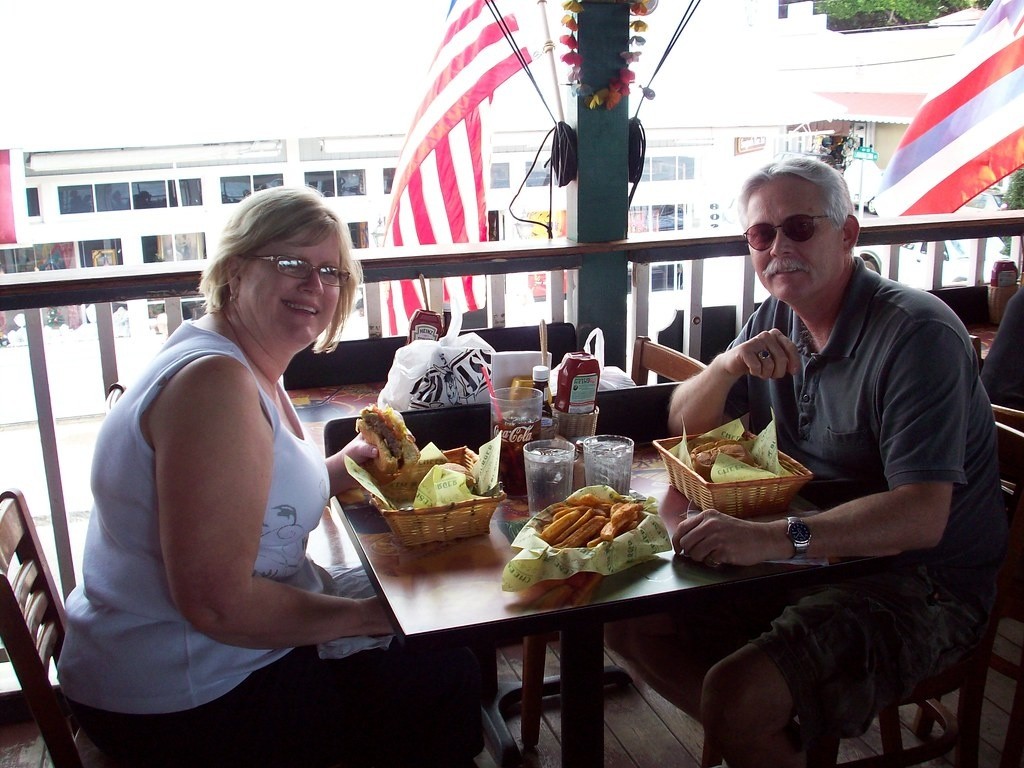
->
[691,438,761,485]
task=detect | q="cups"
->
[583,435,635,497]
[523,439,576,518]
[490,387,543,497]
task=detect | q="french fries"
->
[540,492,645,548]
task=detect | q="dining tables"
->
[273,370,873,767]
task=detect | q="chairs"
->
[1,333,1024,768]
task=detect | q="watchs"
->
[784,515,812,559]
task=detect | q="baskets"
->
[370,446,507,546]
[652,432,814,517]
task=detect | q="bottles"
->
[555,352,600,414]
[532,363,552,419]
[407,310,443,345]
[991,260,1019,286]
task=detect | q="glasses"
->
[242,254,350,287]
[742,213,834,251]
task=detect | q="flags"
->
[873,0,1024,218]
[376,0,532,335]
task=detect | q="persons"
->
[53,184,469,768]
[980,285,1024,412]
[603,153,1003,768]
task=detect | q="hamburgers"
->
[437,462,480,495]
[355,402,421,476]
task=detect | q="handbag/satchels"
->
[549,327,636,397]
[378,299,494,411]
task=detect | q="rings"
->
[707,555,722,567]
[757,349,772,360]
